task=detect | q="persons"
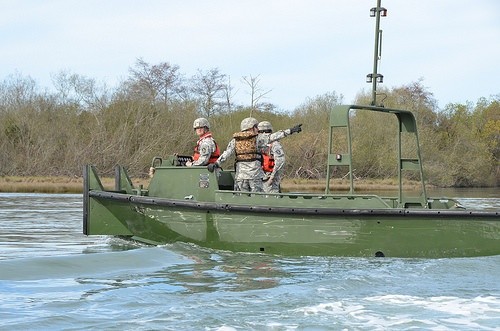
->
[257,120,285,198]
[186,118,220,166]
[207,117,302,197]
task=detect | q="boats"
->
[84,0,500,257]
